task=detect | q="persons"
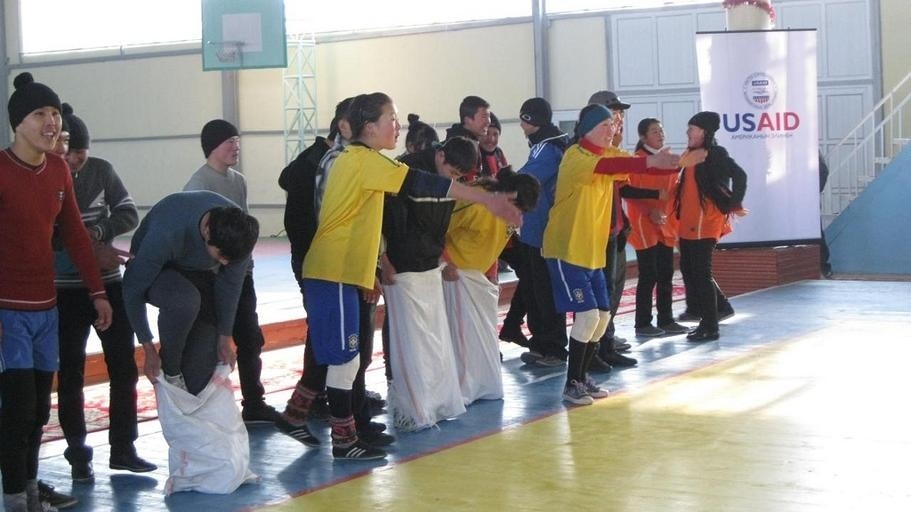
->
[38,121,136,511]
[120,189,261,399]
[275,91,748,461]
[183,117,284,426]
[0,72,115,512]
[817,149,835,280]
[51,102,159,482]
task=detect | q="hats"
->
[688,111,720,139]
[201,119,239,158]
[574,91,631,138]
[520,97,552,127]
[7,72,90,149]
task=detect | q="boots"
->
[588,339,636,372]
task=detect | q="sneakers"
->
[242,402,281,423]
[109,453,157,472]
[38,478,79,510]
[562,381,593,405]
[582,380,608,399]
[499,332,529,347]
[613,336,631,351]
[72,460,94,481]
[520,350,566,367]
[272,390,396,460]
[635,303,735,341]
